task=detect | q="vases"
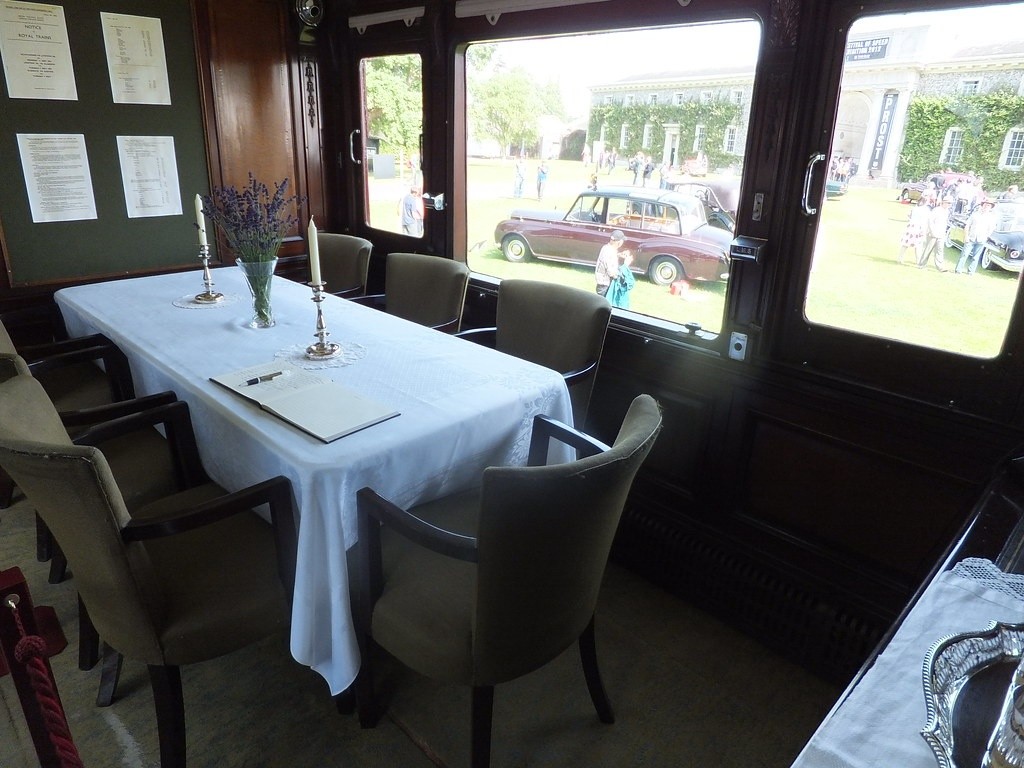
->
[235,255,279,328]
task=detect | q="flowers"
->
[189,168,308,320]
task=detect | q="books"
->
[208,360,402,445]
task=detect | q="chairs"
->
[2,235,663,768]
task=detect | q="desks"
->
[54,268,574,713]
[788,457,1024,768]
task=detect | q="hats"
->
[982,199,995,208]
[610,230,626,241]
[942,195,954,203]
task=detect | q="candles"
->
[195,193,208,249]
[309,214,322,283]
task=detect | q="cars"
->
[494,187,736,286]
[661,159,847,234]
[901,173,1024,272]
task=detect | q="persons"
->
[919,195,955,273]
[536,160,549,199]
[408,153,417,184]
[899,189,936,268]
[826,157,873,182]
[595,230,634,306]
[898,168,1022,221]
[513,156,525,196]
[584,174,598,193]
[581,143,592,168]
[596,149,679,189]
[401,187,424,236]
[955,199,996,274]
